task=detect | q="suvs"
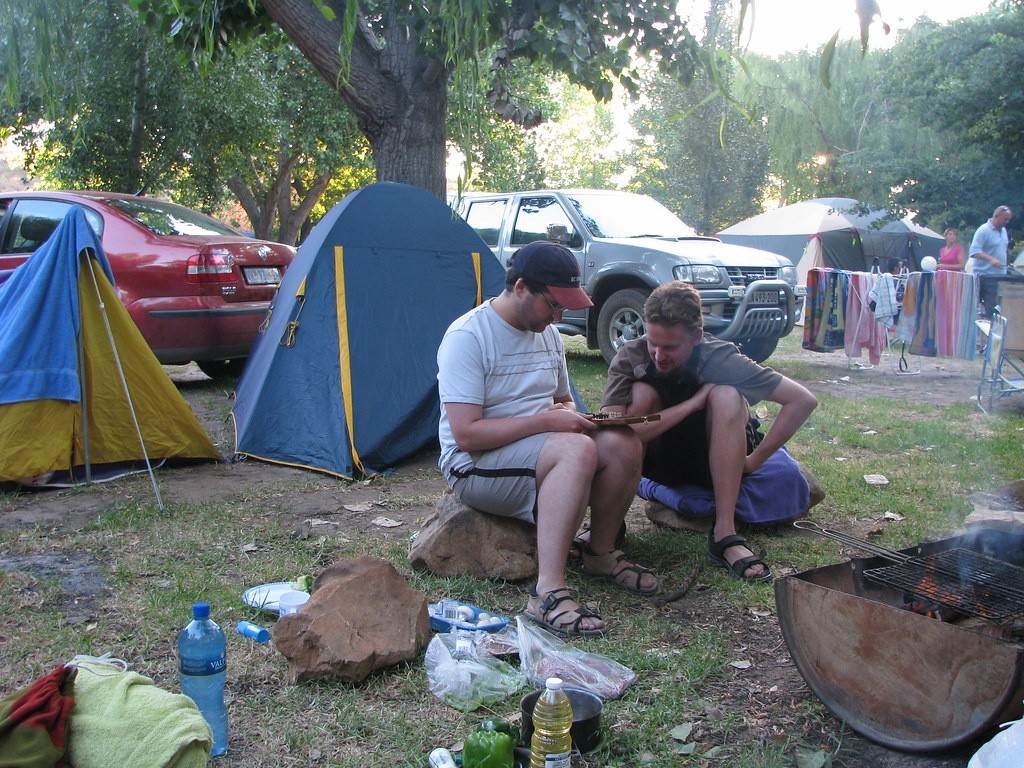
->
[450,189,808,367]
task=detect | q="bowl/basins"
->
[429,597,510,634]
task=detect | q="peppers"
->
[461,718,520,768]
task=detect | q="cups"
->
[279,592,310,618]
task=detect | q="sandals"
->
[580,541,659,596]
[706,526,771,581]
[524,581,607,638]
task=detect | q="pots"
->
[521,690,602,754]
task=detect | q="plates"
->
[243,582,298,610]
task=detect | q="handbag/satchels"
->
[0,663,77,768]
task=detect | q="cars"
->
[0,191,297,379]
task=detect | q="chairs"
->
[972,313,1024,414]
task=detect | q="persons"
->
[887,257,909,327]
[936,226,967,273]
[435,242,660,636]
[964,204,1012,353]
[598,280,821,587]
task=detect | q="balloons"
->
[920,255,937,272]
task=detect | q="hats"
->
[506,239,594,311]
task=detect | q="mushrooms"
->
[457,605,503,628]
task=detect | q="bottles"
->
[239,621,270,642]
[176,604,229,757]
[530,680,573,768]
[428,748,457,768]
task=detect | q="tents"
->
[713,197,946,328]
[0,203,235,518]
[224,180,593,486]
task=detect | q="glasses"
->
[540,291,568,311]
[997,207,1011,213]
